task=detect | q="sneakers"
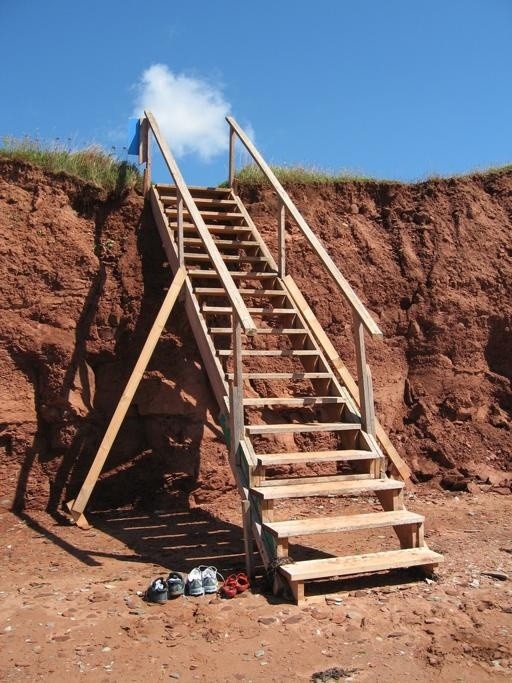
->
[187,566,205,597]
[145,577,170,605]
[203,564,219,594]
[168,572,185,597]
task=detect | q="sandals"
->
[236,572,251,593]
[222,573,237,598]
